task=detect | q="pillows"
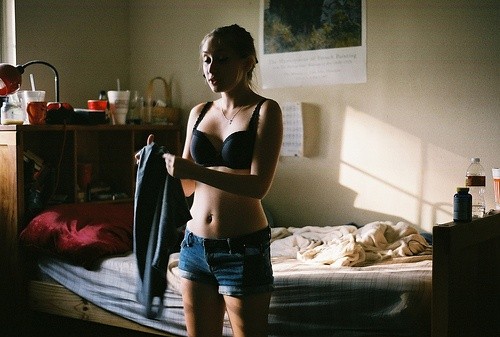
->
[16,200,132,266]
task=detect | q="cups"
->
[26,101,47,126]
[492,168,500,208]
[23,90,45,125]
[144,94,156,124]
[87,100,108,110]
[107,91,130,125]
[78,162,93,192]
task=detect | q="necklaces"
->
[222,107,242,124]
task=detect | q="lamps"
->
[0,60,75,124]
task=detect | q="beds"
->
[20,235,434,337]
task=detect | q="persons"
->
[136,25,284,337]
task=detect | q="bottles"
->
[126,87,144,126]
[87,183,91,202]
[466,158,486,218]
[454,187,472,223]
[0,102,24,125]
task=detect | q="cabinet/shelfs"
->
[431,217,500,337]
[0,124,182,256]
